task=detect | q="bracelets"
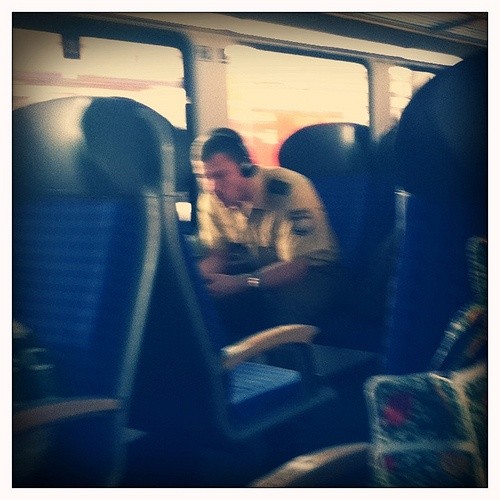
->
[248,270,262,288]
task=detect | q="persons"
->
[191,126,337,477]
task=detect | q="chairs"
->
[239,51,487,487]
[131,106,344,488]
[13,97,163,489]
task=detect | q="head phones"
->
[207,131,260,178]
